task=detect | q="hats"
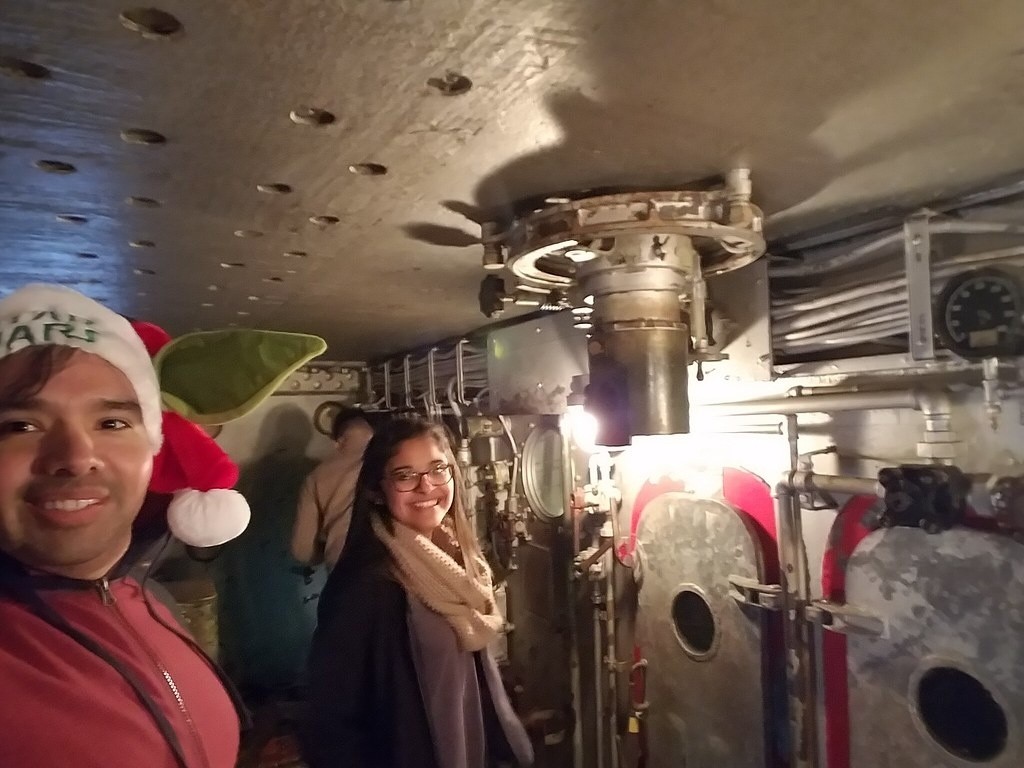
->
[0,282,327,457]
[119,314,251,548]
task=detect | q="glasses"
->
[384,464,455,492]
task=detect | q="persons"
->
[306,420,534,768]
[289,407,374,570]
[0,281,264,768]
